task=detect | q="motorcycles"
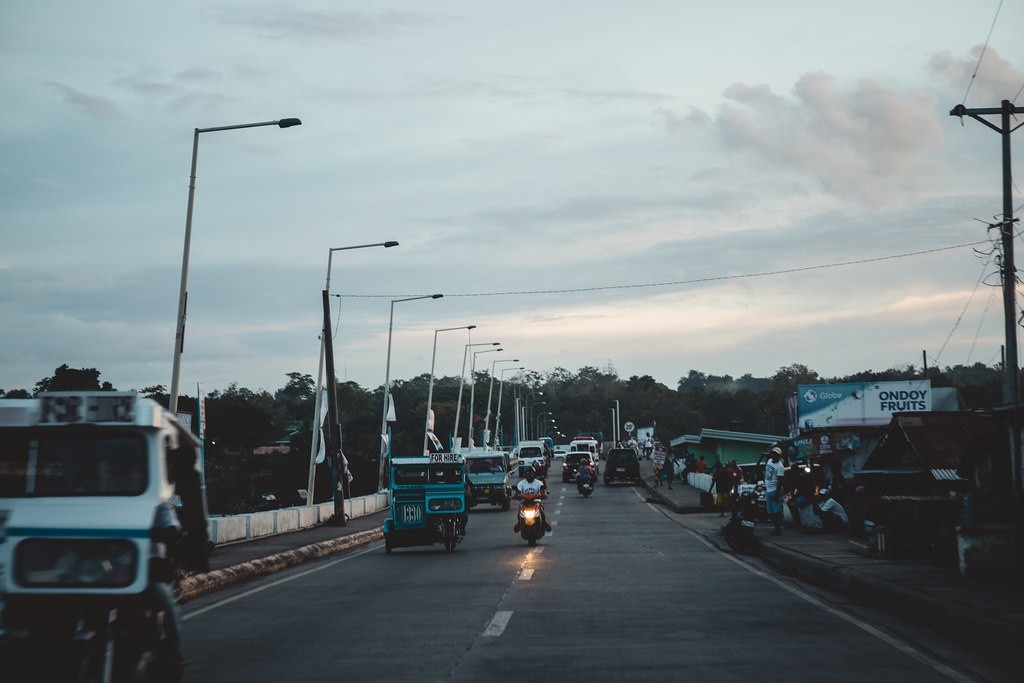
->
[511,485,552,547]
[383,452,478,554]
[0,385,215,683]
[573,463,593,498]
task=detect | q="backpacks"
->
[645,438,652,447]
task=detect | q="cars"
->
[730,462,768,501]
[554,450,567,459]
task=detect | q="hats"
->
[772,446,782,455]
[819,488,830,495]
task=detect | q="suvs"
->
[604,448,641,488]
[562,452,598,483]
[464,451,512,511]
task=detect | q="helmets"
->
[524,466,537,473]
[581,458,587,464]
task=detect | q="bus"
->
[538,436,554,459]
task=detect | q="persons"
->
[681,453,696,485]
[644,433,654,459]
[653,449,679,489]
[808,488,847,533]
[709,461,743,517]
[787,465,814,527]
[765,447,786,535]
[514,466,551,532]
[577,458,594,493]
[695,455,709,474]
[628,437,637,445]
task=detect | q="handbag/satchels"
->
[700,492,714,507]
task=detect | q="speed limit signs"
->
[624,421,635,432]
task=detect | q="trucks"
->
[570,437,600,462]
[577,431,604,453]
[516,440,550,479]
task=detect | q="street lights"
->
[157,116,303,412]
[376,287,568,504]
[608,399,621,449]
[303,239,400,507]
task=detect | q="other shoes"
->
[545,524,551,531]
[769,529,781,536]
[514,524,518,533]
[668,486,672,490]
